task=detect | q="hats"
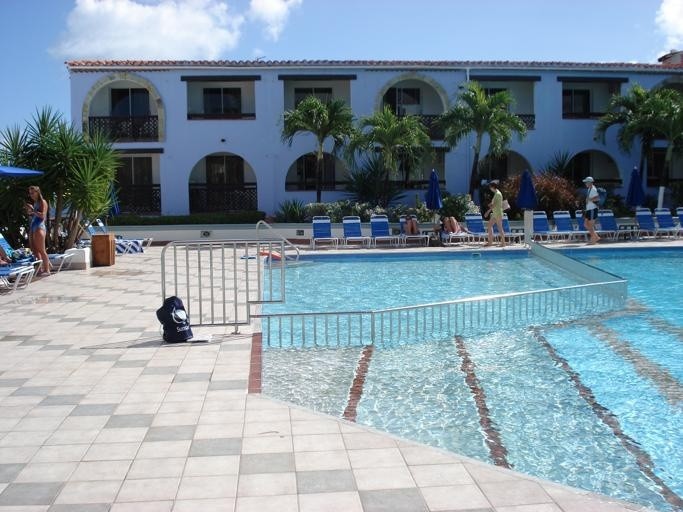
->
[582,176,594,183]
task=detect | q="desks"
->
[619,224,640,242]
[510,228,525,243]
[422,229,438,247]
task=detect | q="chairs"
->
[492,212,526,242]
[465,213,492,245]
[399,215,430,248]
[634,208,674,242]
[371,214,399,247]
[595,209,635,242]
[0,218,153,289]
[313,216,339,248]
[574,210,617,243]
[529,210,564,244]
[655,208,683,240]
[552,210,590,244]
[342,215,371,247]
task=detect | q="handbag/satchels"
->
[502,199,510,211]
[597,188,608,206]
[156,296,193,343]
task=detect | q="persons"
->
[406,218,418,234]
[484,183,506,246]
[0,185,54,278]
[441,215,466,234]
[581,177,607,243]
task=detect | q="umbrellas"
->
[625,166,646,206]
[101,179,119,235]
[515,169,538,210]
[425,169,443,210]
[0,163,44,180]
